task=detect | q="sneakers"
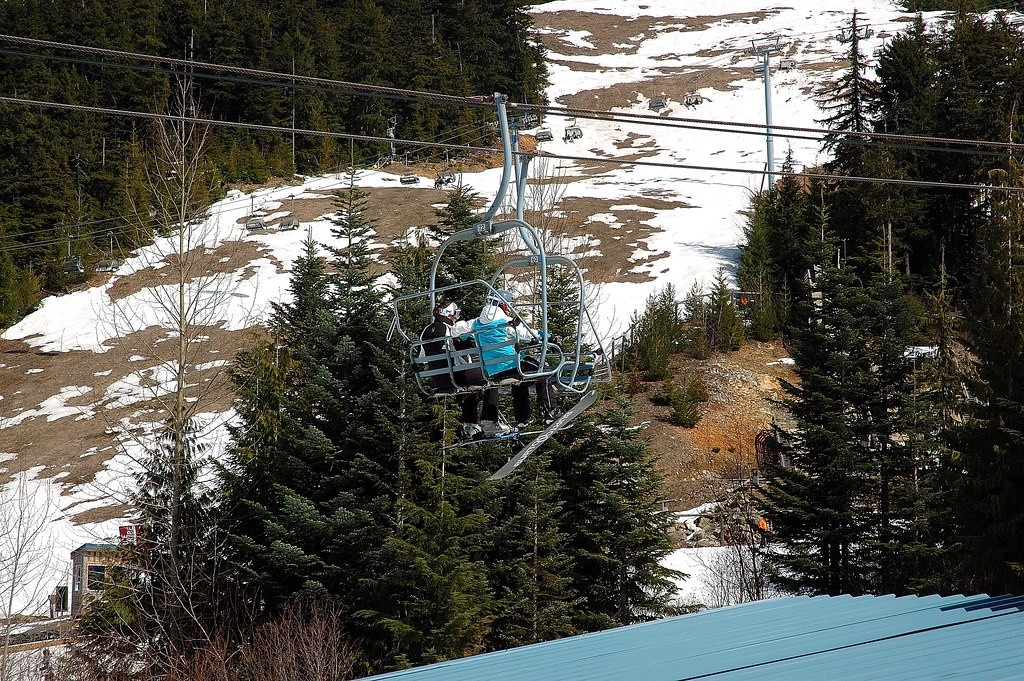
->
[543,406,575,430]
[517,417,535,427]
[480,419,511,438]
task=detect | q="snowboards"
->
[485,389,598,482]
[438,423,575,450]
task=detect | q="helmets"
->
[488,290,514,302]
[433,301,461,326]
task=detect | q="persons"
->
[450,290,574,430]
[418,298,511,438]
